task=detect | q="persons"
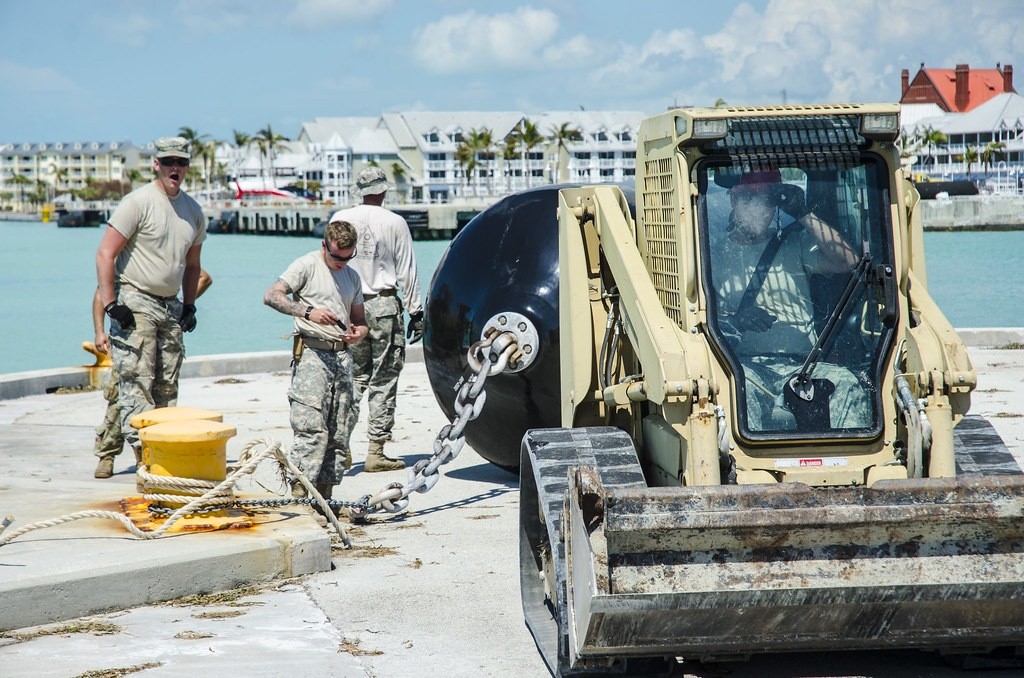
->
[93,137,214,479]
[262,220,371,526]
[330,167,425,472]
[713,163,872,434]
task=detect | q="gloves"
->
[407,317,424,344]
[718,305,777,333]
[769,184,813,220]
[179,304,197,332]
[105,302,135,330]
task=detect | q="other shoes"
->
[94,455,113,478]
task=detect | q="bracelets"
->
[305,306,313,320]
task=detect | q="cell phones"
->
[771,189,794,205]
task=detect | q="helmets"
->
[729,164,783,192]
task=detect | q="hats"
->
[348,166,395,199]
[155,137,191,159]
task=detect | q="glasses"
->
[326,242,357,260]
[158,158,190,166]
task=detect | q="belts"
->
[380,289,396,296]
[303,338,346,351]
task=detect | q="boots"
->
[292,482,328,527]
[364,441,406,472]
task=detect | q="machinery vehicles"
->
[517,103,1024,678]
